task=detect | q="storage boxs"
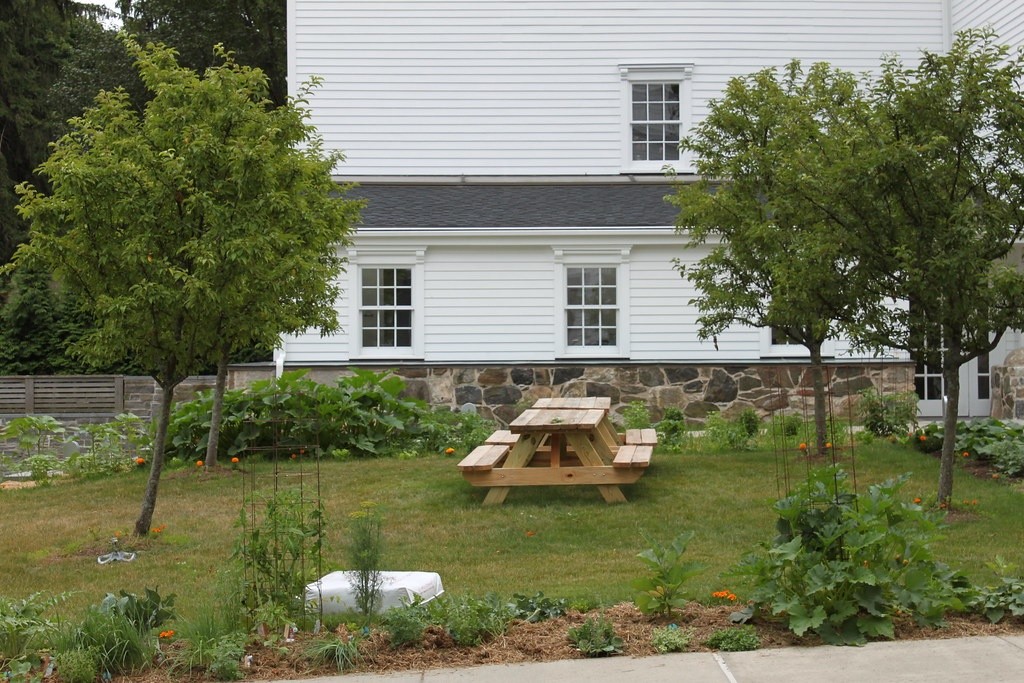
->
[304,570,445,616]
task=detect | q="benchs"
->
[626,429,658,447]
[456,445,510,471]
[484,429,522,450]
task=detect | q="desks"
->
[613,445,653,468]
[531,396,625,452]
[462,409,644,506]
